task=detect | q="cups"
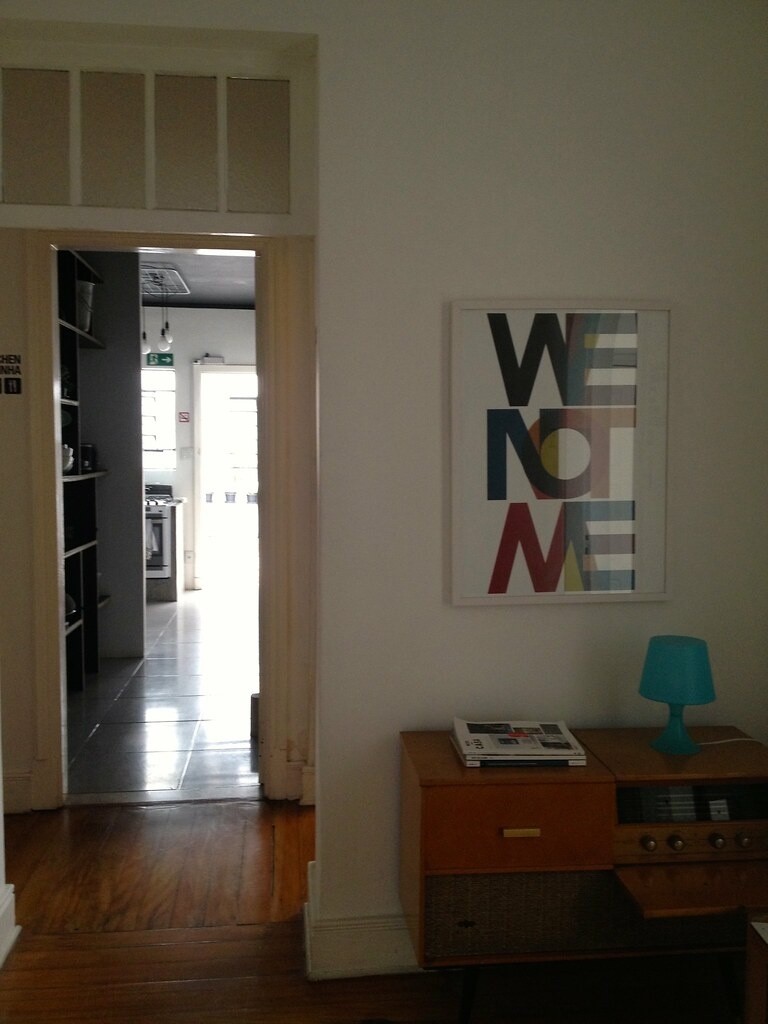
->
[81,444,96,474]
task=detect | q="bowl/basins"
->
[62,445,74,475]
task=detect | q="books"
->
[448,718,586,766]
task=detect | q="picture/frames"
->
[447,299,676,608]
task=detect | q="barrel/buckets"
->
[76,280,95,331]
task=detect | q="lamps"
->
[639,634,715,755]
[140,277,173,354]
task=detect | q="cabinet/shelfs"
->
[399,725,768,985]
[61,249,110,695]
